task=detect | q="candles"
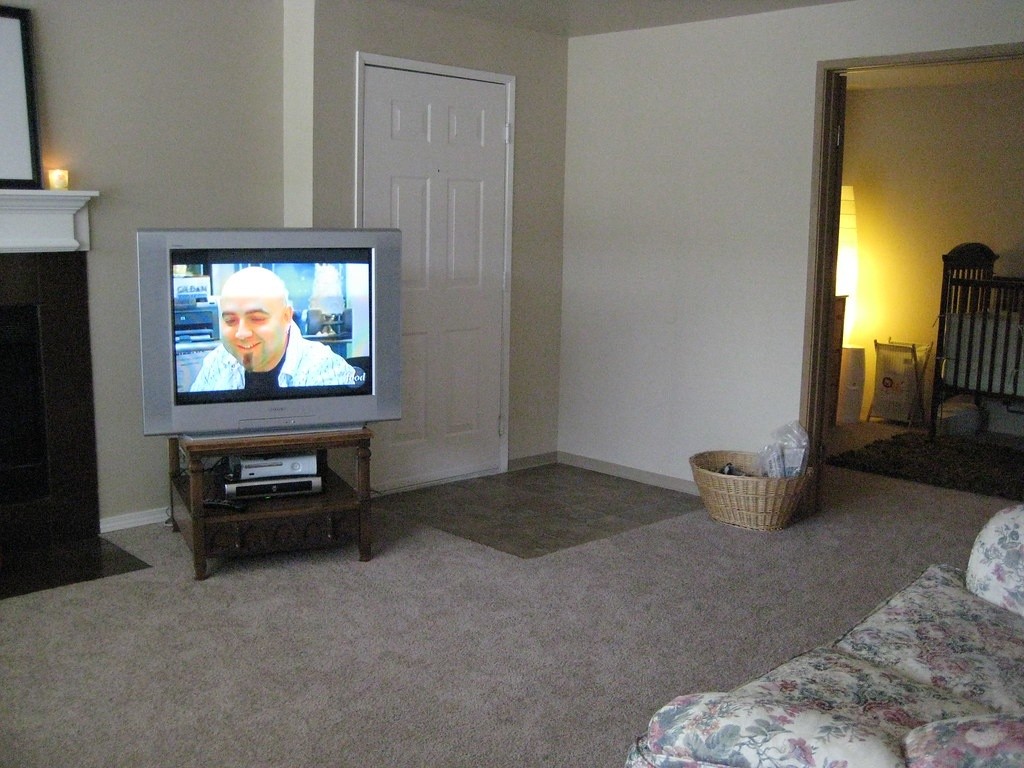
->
[49,169,69,191]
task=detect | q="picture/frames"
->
[0,5,44,189]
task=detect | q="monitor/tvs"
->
[136,227,402,441]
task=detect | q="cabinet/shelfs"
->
[823,294,850,435]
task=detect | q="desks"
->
[170,426,376,581]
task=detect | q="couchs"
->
[621,505,1024,768]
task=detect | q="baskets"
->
[689,450,813,533]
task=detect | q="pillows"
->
[901,712,1024,768]
[647,693,905,768]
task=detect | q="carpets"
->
[370,462,704,559]
[825,428,1024,503]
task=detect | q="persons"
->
[189,266,357,392]
